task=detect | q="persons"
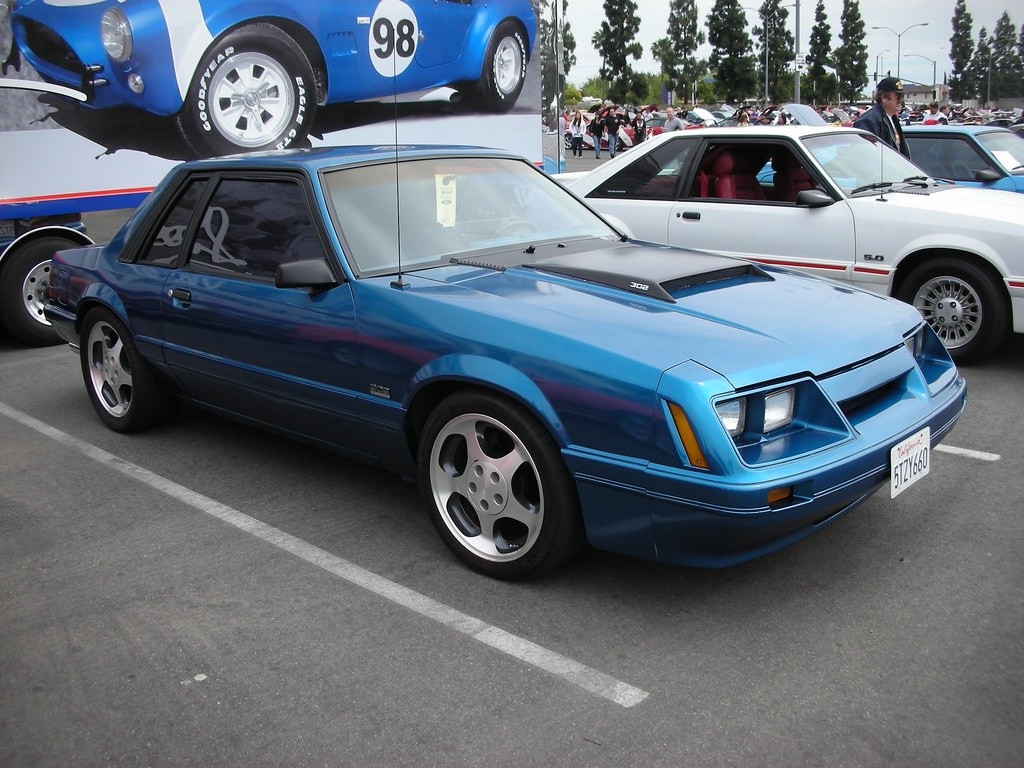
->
[568,78,1024,163]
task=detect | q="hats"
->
[929,102,939,108]
[876,76,909,94]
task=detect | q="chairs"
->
[713,149,767,202]
[914,145,950,179]
[944,142,975,180]
[772,154,811,202]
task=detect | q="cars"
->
[39,146,968,582]
[748,103,1024,196]
[557,104,1024,150]
[503,127,1024,363]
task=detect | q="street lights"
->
[872,23,929,80]
[963,38,1017,110]
[902,54,936,103]
[875,49,891,88]
[734,3,802,109]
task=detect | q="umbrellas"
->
[600,105,619,115]
[588,104,601,113]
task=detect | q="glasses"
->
[894,94,906,100]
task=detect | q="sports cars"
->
[12,0,536,154]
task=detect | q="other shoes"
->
[573,156,578,159]
[596,156,600,159]
[579,157,583,159]
[611,154,614,159]
